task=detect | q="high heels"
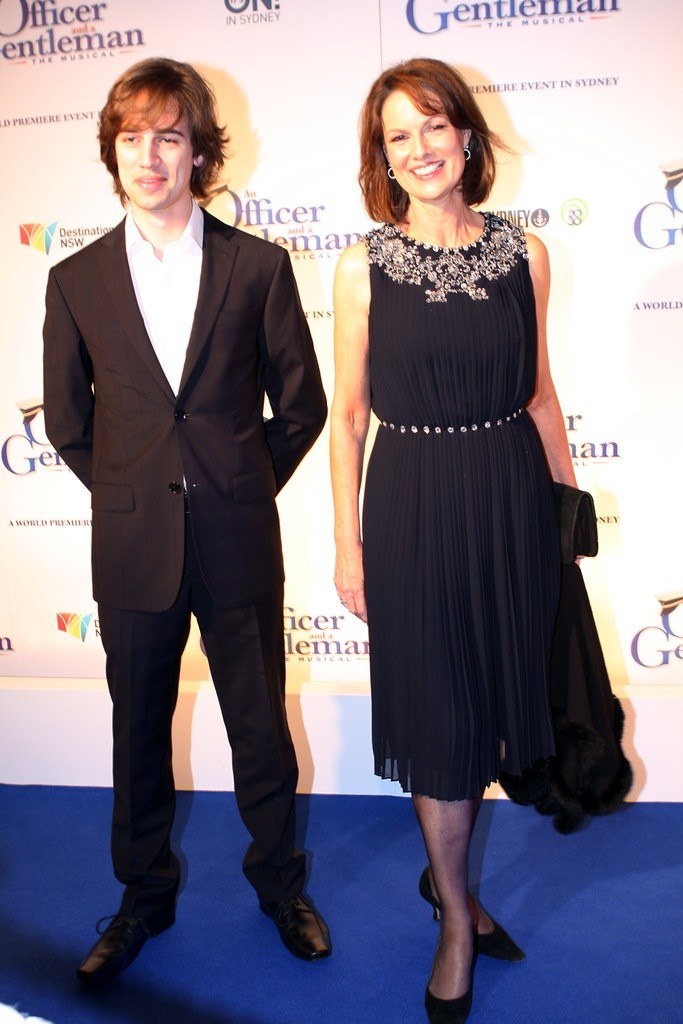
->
[419,866,526,962]
[424,930,476,1024]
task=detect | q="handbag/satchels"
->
[553,480,597,566]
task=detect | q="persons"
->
[331,57,634,1024]
[43,58,330,980]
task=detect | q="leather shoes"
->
[260,896,332,961]
[76,914,148,986]
